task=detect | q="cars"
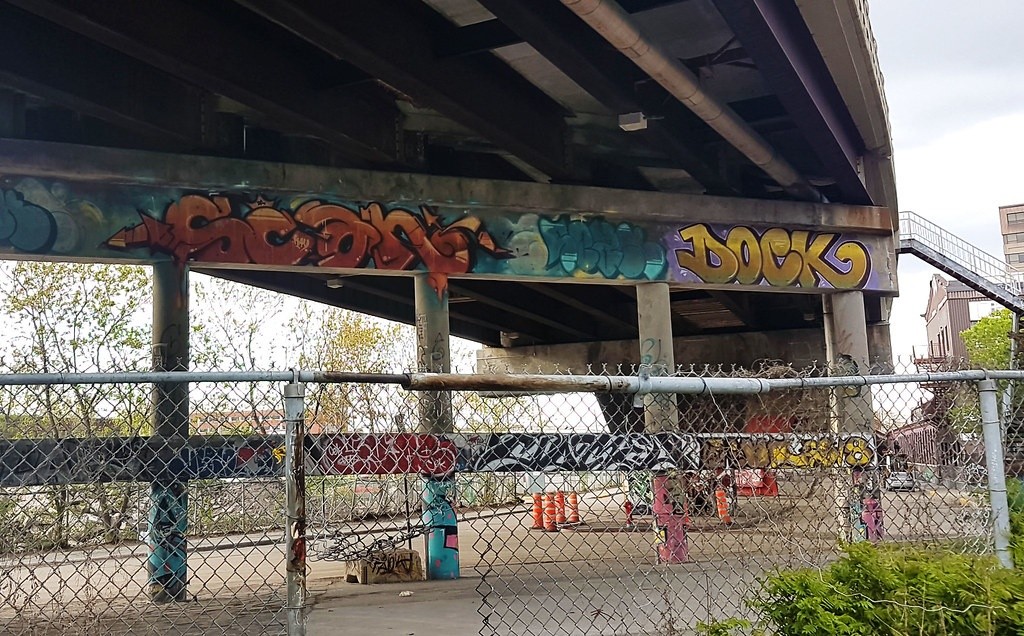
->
[884,472,915,491]
